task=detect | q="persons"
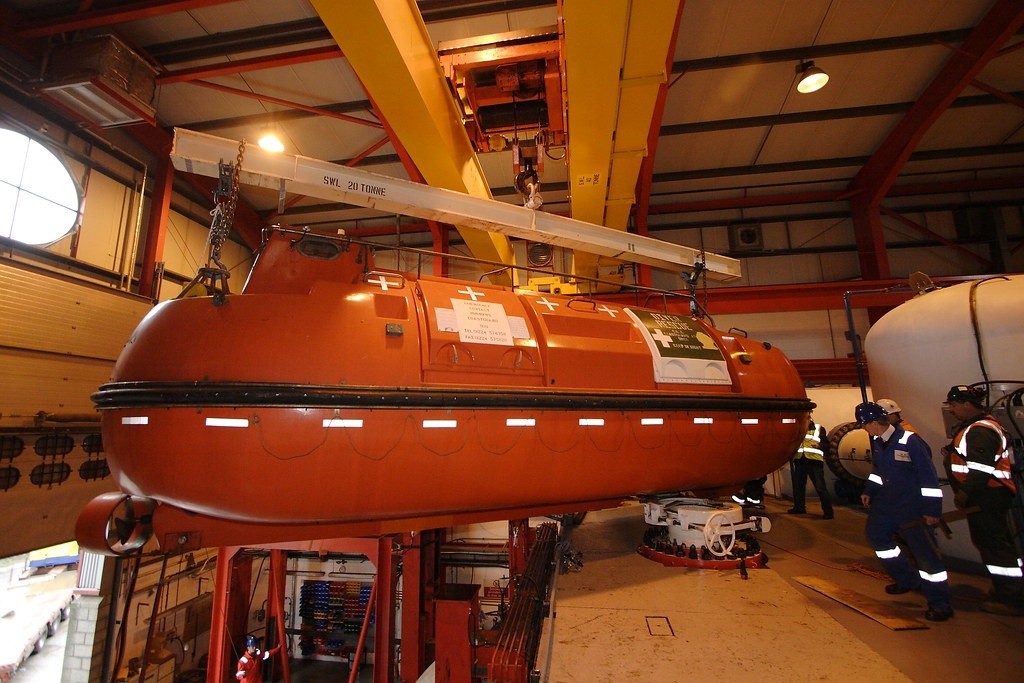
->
[786,412,835,521]
[730,476,769,512]
[873,398,940,595]
[854,401,956,621]
[943,385,1024,614]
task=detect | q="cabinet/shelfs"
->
[117,649,176,683]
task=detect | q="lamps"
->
[795,60,829,94]
[328,559,376,580]
[263,558,326,577]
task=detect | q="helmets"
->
[876,399,901,415]
[942,385,987,405]
[246,636,258,647]
[854,401,888,425]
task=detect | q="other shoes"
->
[885,581,912,594]
[824,512,833,520]
[924,603,952,621]
[978,596,1024,618]
[788,506,806,515]
